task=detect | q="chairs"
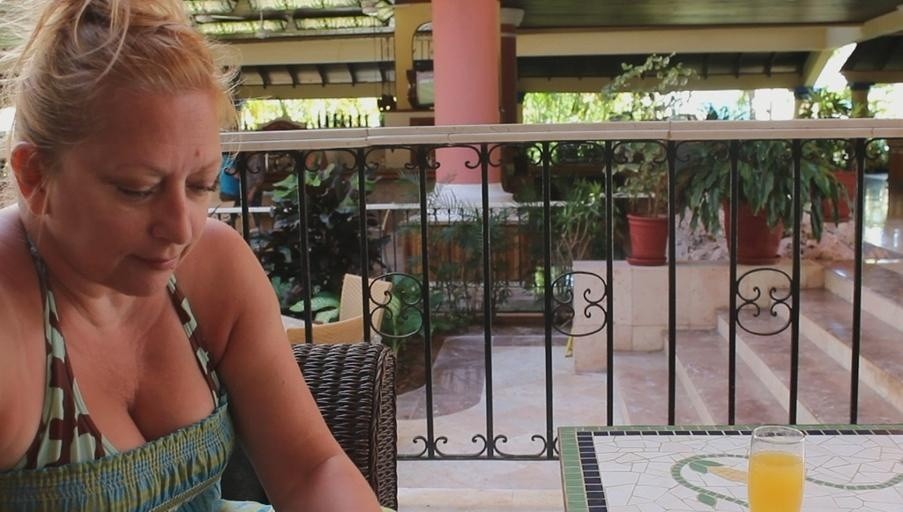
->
[220,345,399,511]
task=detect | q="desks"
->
[556,422,903,512]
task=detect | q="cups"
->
[749,426,804,512]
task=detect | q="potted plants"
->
[677,108,823,265]
[803,81,888,223]
[574,58,700,267]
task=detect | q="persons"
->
[226,153,267,229]
[218,150,240,206]
[1,1,394,512]
